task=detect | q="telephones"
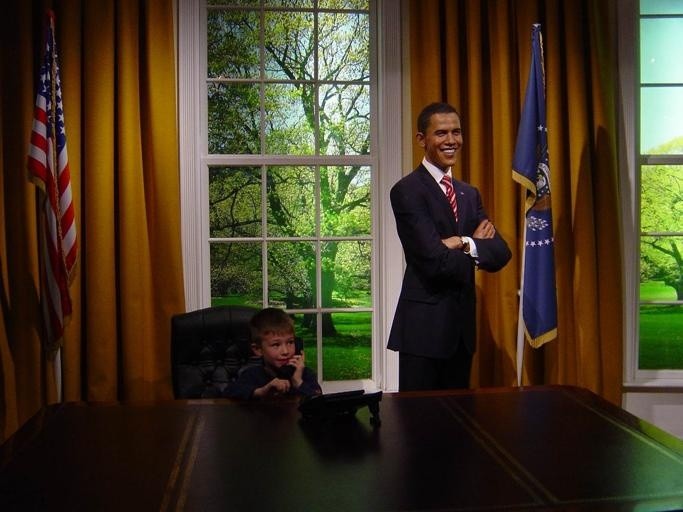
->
[278,337,303,378]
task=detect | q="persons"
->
[223,306,321,399]
[382,103,510,392]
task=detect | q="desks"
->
[0,383,682,512]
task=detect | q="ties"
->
[440,176,458,221]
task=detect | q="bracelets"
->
[458,235,467,251]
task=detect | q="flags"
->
[20,13,79,367]
[510,31,558,351]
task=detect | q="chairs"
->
[168,305,297,399]
